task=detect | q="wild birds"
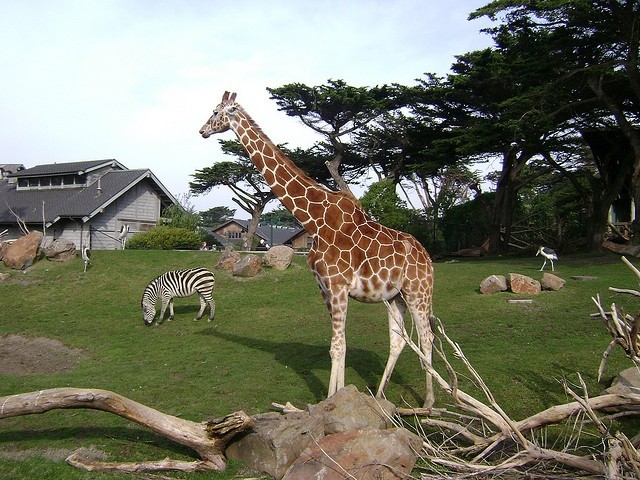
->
[118,224,130,250]
[82,244,91,272]
[535,246,559,272]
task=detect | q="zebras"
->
[141,267,216,327]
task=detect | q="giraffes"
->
[199,90,436,411]
